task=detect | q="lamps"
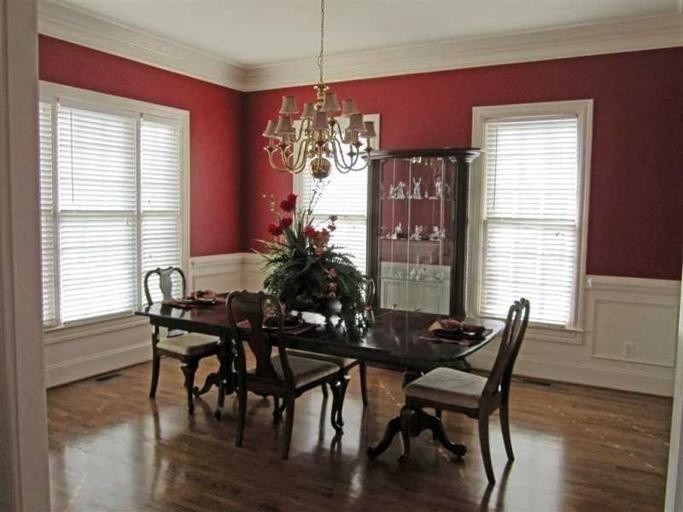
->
[263,1,377,179]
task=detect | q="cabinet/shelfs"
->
[367,148,479,320]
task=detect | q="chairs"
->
[226,289,343,460]
[400,298,530,485]
[272,274,375,417]
[144,267,234,415]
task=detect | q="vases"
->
[280,290,365,342]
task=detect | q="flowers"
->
[251,177,373,332]
